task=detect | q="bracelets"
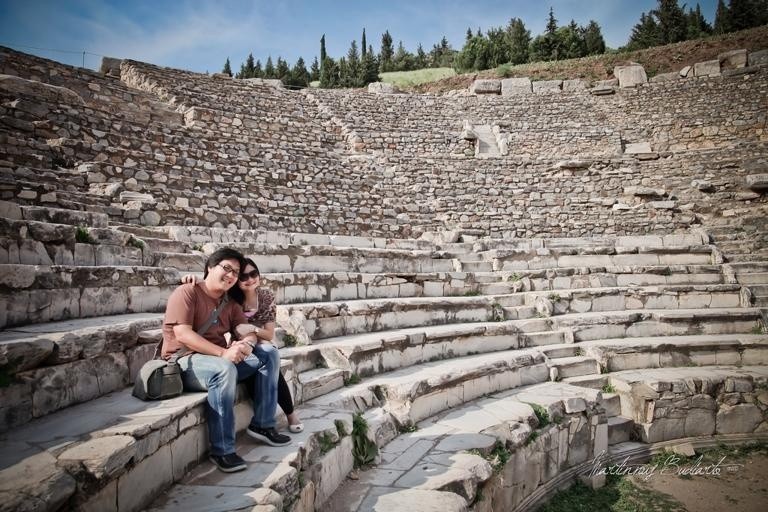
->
[246,342,255,348]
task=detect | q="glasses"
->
[218,263,240,278]
[238,269,260,282]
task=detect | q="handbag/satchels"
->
[131,359,183,402]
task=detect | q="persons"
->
[182,259,303,433]
[161,249,292,474]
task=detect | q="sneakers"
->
[209,453,246,473]
[247,424,291,446]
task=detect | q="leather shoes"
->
[287,420,303,433]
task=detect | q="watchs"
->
[253,326,259,336]
